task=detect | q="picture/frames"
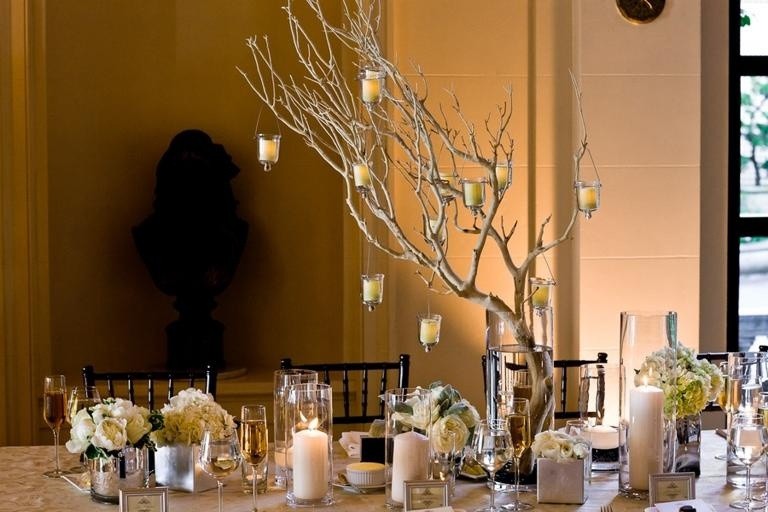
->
[647,471,696,508]
[118,487,169,512]
[403,481,450,511]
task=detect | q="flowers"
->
[391,382,482,453]
[633,346,725,420]
[531,430,589,459]
[148,387,235,449]
[66,398,152,455]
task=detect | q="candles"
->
[362,279,382,304]
[491,167,508,189]
[391,431,432,507]
[355,164,372,185]
[421,320,439,346]
[362,78,380,104]
[629,384,664,492]
[464,182,484,207]
[290,433,329,501]
[259,138,277,163]
[533,284,550,309]
[578,187,598,211]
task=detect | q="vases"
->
[414,432,457,495]
[89,449,145,504]
[659,413,676,473]
[156,441,233,492]
[537,456,592,505]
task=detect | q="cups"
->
[197,425,245,512]
[66,386,102,472]
[41,375,67,479]
[501,398,536,512]
[732,414,765,512]
[619,312,677,501]
[272,370,318,490]
[241,465,266,495]
[241,404,262,512]
[469,419,514,512]
[578,362,619,471]
[430,430,452,492]
[485,307,555,491]
[758,392,768,497]
[565,420,592,444]
[383,388,434,511]
[715,361,727,460]
[282,384,335,508]
[726,351,768,489]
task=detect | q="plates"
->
[332,482,392,492]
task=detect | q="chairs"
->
[677,344,768,410]
[281,355,410,436]
[481,353,608,436]
[83,366,220,412]
